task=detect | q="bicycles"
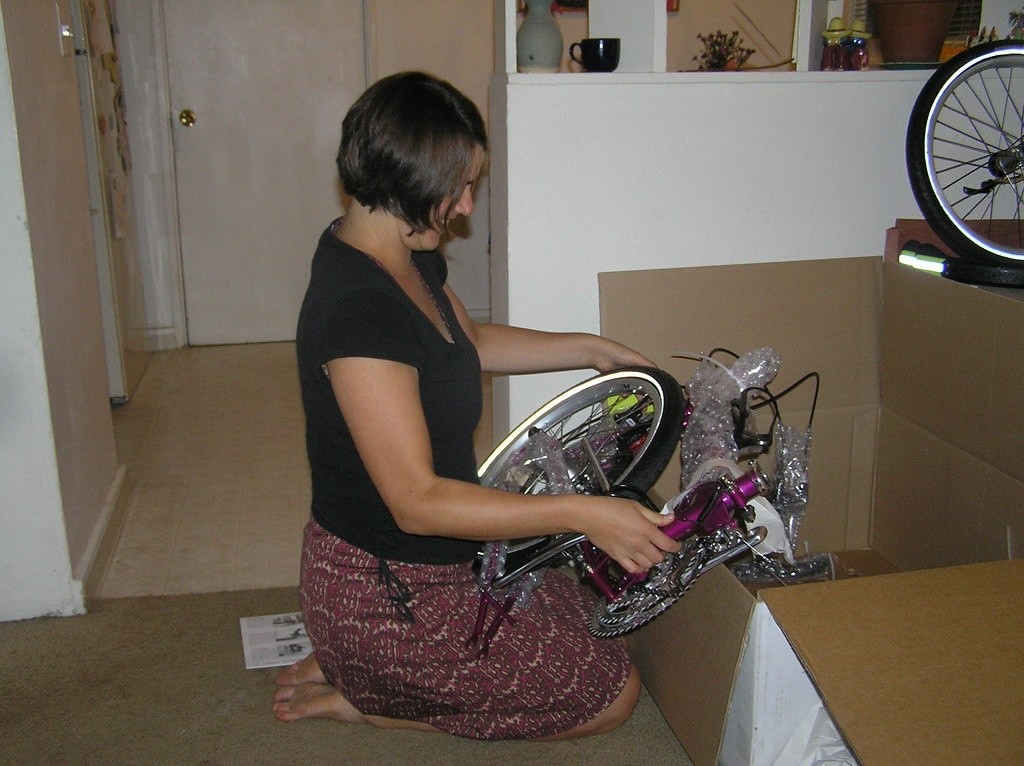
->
[905,39,1024,271]
[467,349,834,659]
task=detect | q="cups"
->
[569,38,621,72]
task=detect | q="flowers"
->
[690,29,755,71]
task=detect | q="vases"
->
[869,0,958,63]
[517,0,564,73]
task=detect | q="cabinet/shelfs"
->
[487,0,1024,455]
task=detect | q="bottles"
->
[820,17,873,71]
[517,0,563,73]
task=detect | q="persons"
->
[271,70,684,741]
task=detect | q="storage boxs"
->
[596,255,1024,766]
